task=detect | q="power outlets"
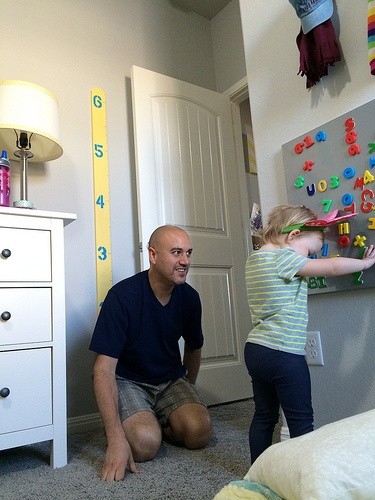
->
[304,331,324,366]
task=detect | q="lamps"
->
[0,80,64,208]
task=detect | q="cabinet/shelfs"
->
[0,206,77,470]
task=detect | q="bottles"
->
[0,150,11,207]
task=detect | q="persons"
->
[88,225,214,482]
[244,206,375,467]
